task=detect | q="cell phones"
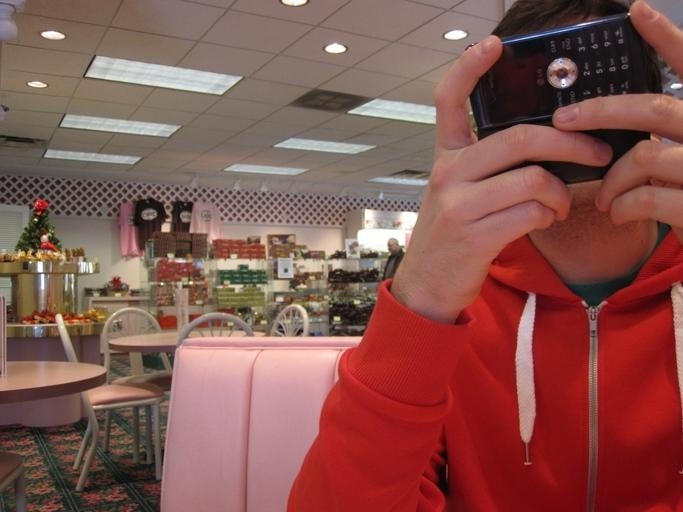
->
[465,10,666,183]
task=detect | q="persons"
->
[382,236,405,281]
[285,1,683,512]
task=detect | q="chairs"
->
[176,311,254,347]
[272,303,309,338]
[56,312,160,492]
[101,307,173,465]
[158,336,366,512]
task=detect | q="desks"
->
[0,360,107,512]
[109,329,271,467]
